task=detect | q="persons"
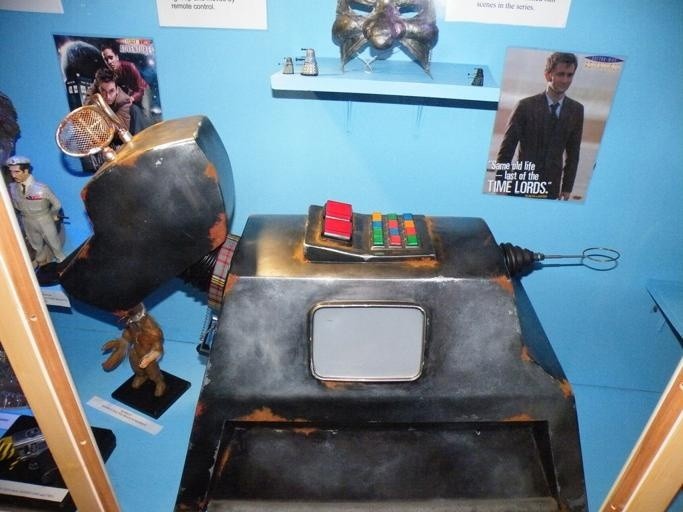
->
[83,70,142,159]
[496,51,585,200]
[3,155,65,267]
[94,39,151,121]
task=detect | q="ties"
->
[549,102,560,132]
